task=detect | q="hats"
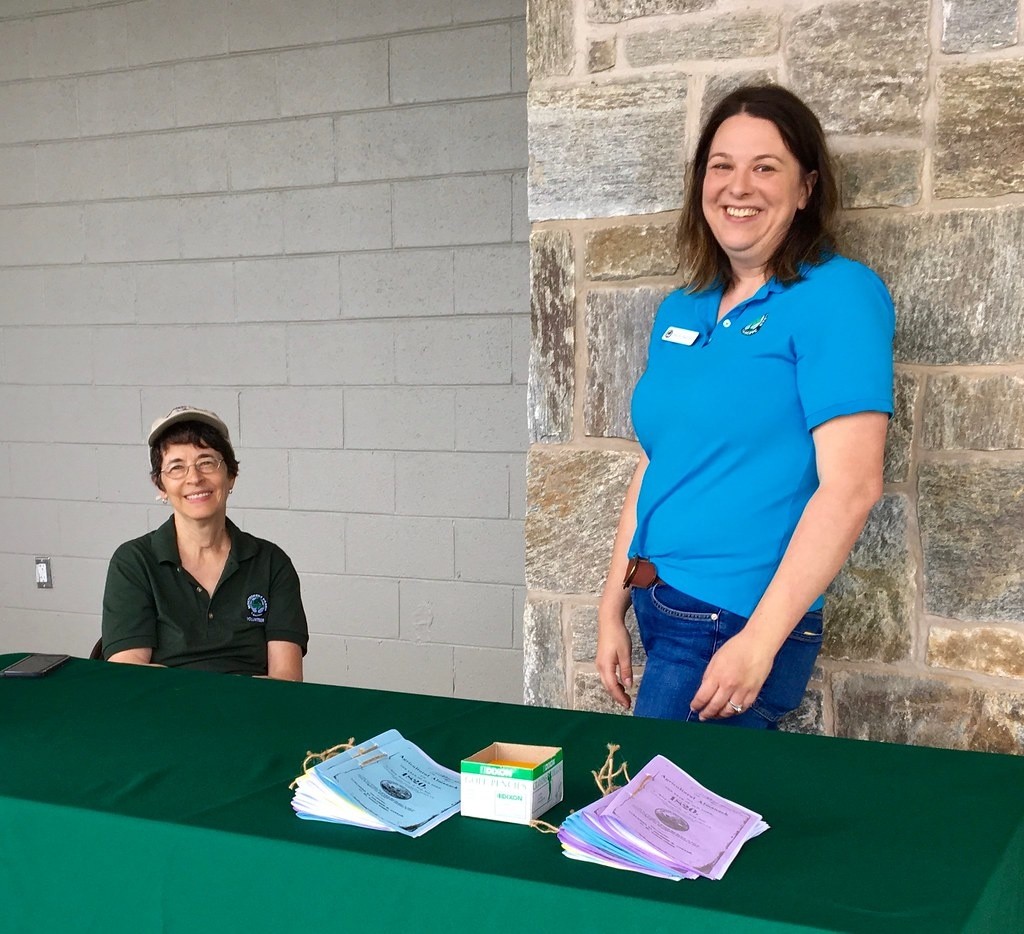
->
[147,405,230,448]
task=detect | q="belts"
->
[622,552,668,590]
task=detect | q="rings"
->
[728,700,743,713]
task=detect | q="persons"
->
[593,82,897,730]
[101,405,308,682]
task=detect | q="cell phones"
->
[0,655,71,678]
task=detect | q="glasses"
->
[160,457,225,479]
